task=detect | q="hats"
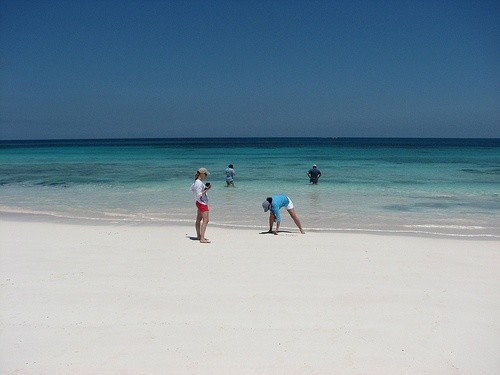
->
[262,201,269,212]
[313,164,317,166]
[197,168,210,174]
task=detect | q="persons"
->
[307,164,321,184]
[262,194,305,235]
[190,167,212,243]
[225,164,236,187]
